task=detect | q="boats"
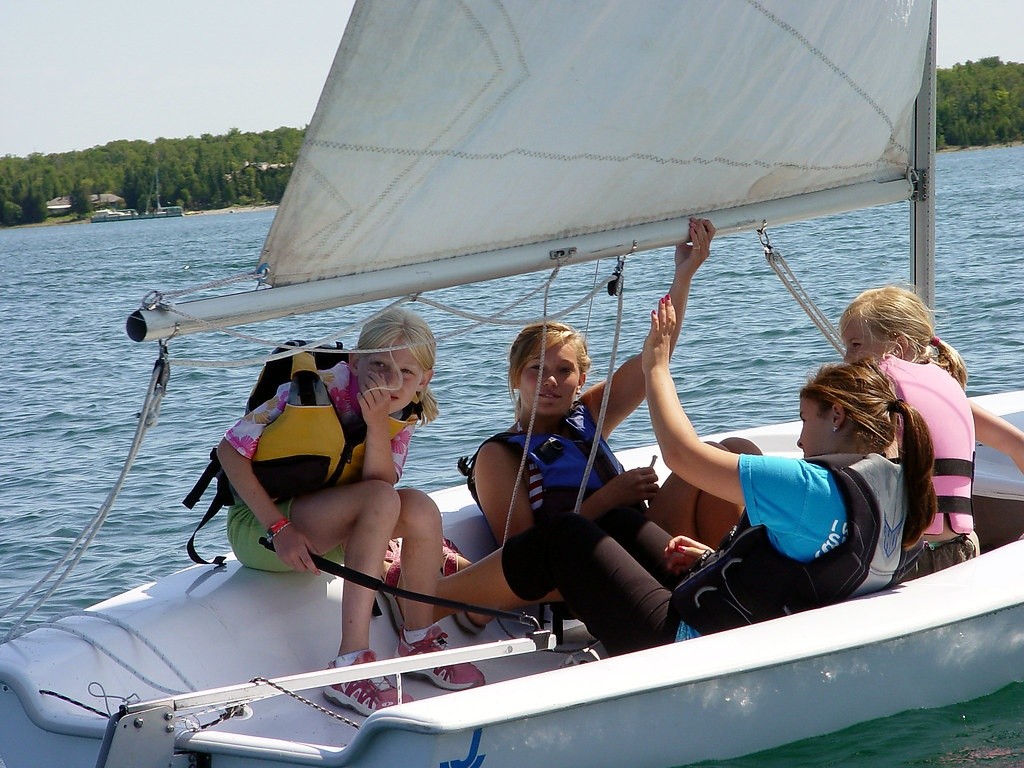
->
[90,166,168,224]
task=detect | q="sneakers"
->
[395,625,486,688]
[322,649,415,717]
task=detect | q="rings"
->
[693,245,701,249]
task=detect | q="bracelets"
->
[267,518,292,543]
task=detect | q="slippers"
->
[380,540,406,630]
[441,538,485,634]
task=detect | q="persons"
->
[456,217,762,645]
[375,295,937,658]
[217,306,486,716]
[839,287,1024,586]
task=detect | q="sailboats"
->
[0,1,1024,768]
